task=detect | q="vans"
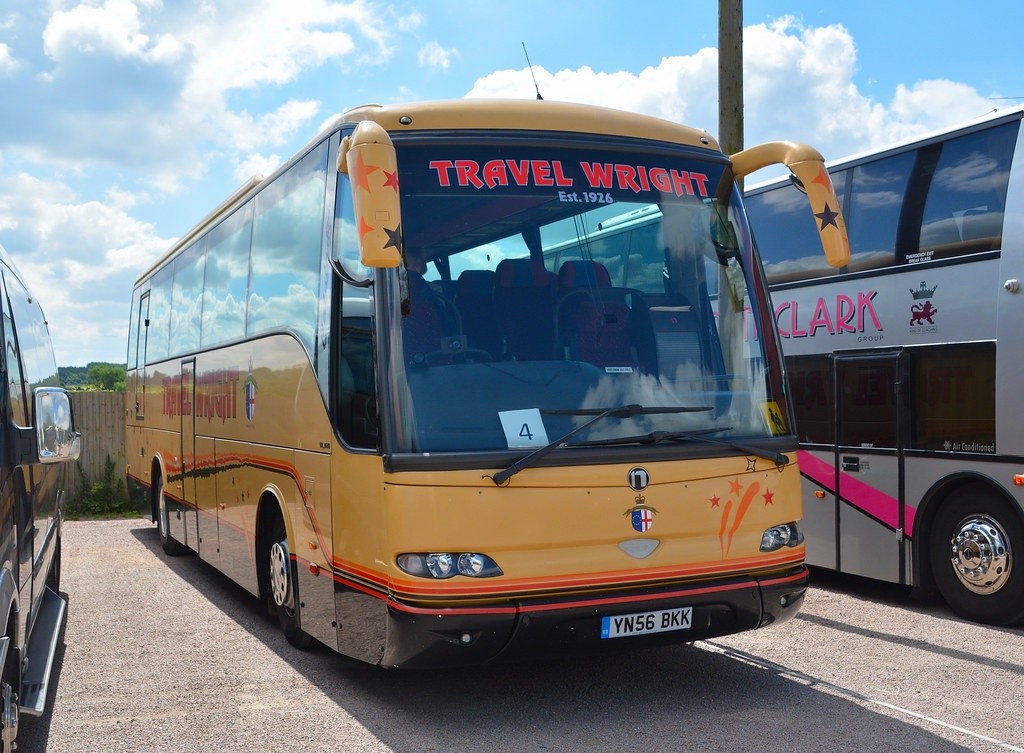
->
[1,248,81,753]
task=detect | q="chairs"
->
[427,259,732,419]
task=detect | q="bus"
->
[123,103,850,670]
[516,103,1024,622]
